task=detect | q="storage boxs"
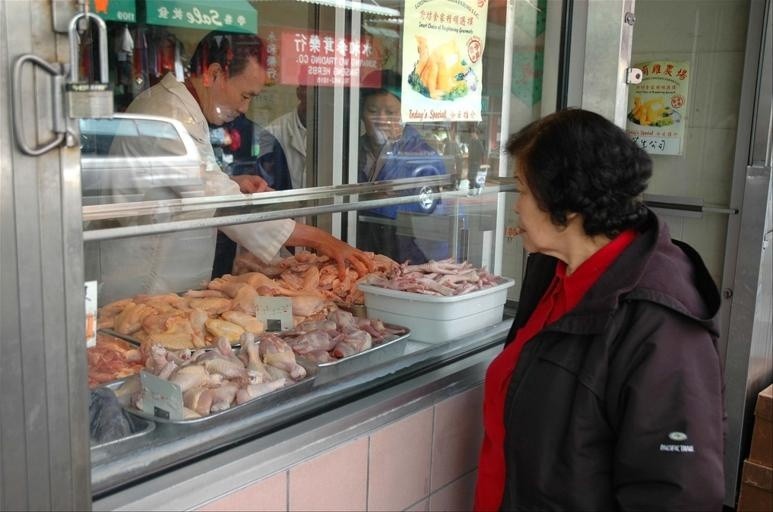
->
[356,265,516,346]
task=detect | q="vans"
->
[78,112,208,234]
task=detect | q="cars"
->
[360,123,457,215]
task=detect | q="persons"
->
[85,22,374,307]
[206,111,292,282]
[236,58,308,267]
[470,108,728,511]
[355,68,449,270]
